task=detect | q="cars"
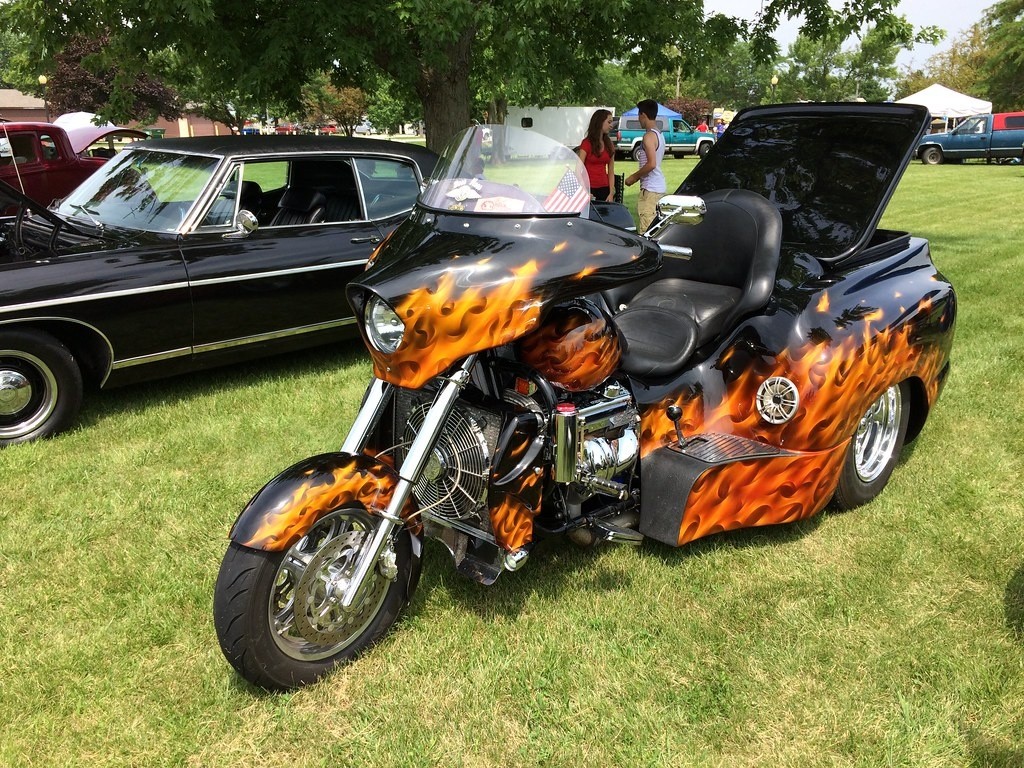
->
[0,133,637,451]
[0,121,148,216]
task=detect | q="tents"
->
[896,83,993,132]
[623,103,682,119]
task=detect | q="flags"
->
[542,168,591,217]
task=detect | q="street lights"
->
[39,74,49,123]
[771,75,778,104]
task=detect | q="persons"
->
[625,98,667,234]
[578,109,616,201]
[715,119,726,139]
[696,120,709,132]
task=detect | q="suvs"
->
[609,116,715,162]
[355,122,377,136]
[275,122,298,135]
[914,112,1024,165]
[320,125,336,133]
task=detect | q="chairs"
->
[265,185,328,226]
[207,180,262,225]
[592,187,782,383]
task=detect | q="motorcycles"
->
[212,96,957,693]
